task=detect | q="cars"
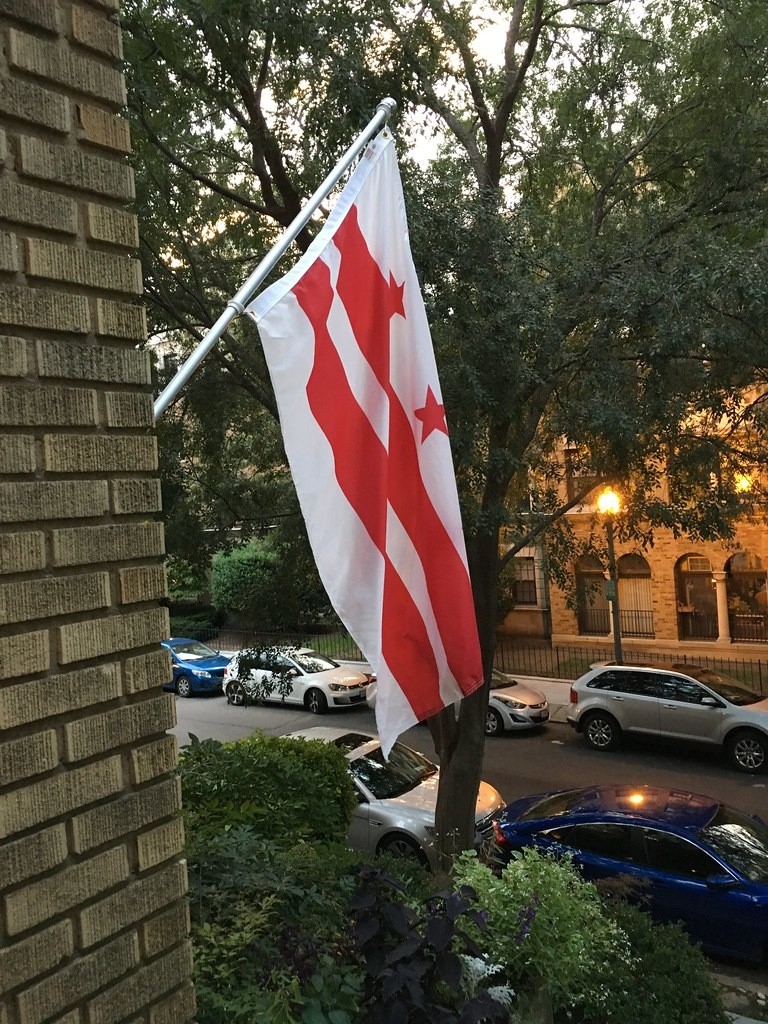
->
[280,727,507,879]
[482,784,767,965]
[366,668,551,737]
[222,645,369,714]
[159,638,231,698]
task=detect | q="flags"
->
[242,121,484,763]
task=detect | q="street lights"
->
[597,486,622,661]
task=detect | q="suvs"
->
[566,658,768,775]
